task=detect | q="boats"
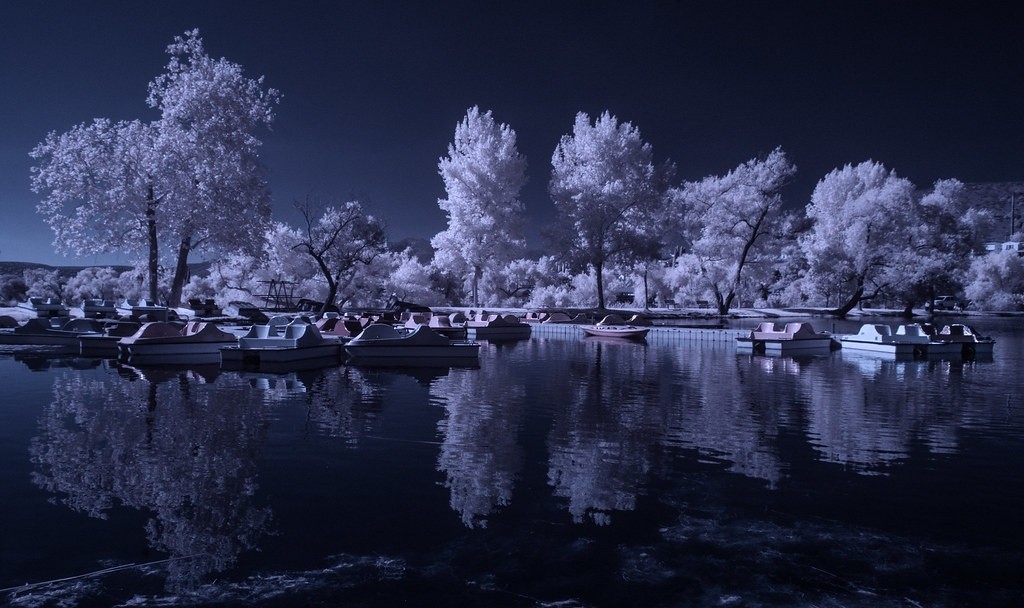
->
[0,295,610,371]
[838,322,997,355]
[734,321,834,352]
[581,314,651,342]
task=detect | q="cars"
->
[925,295,968,311]
